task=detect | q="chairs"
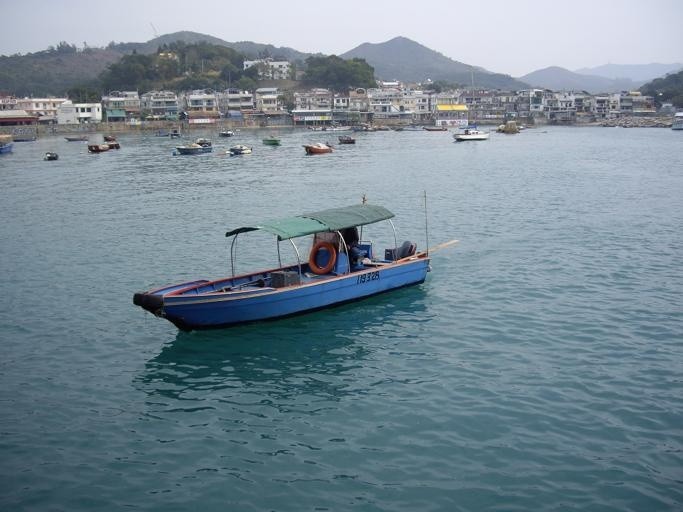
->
[355,243,373,269]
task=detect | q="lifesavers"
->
[309,241,337,274]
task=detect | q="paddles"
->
[379,240,459,264]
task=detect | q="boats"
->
[229,145,252,155]
[217,130,235,137]
[452,128,489,140]
[422,125,448,132]
[671,112,683,130]
[261,135,281,146]
[338,135,355,144]
[301,142,332,156]
[128,200,431,332]
[0,125,213,162]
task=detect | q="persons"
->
[342,227,359,264]
